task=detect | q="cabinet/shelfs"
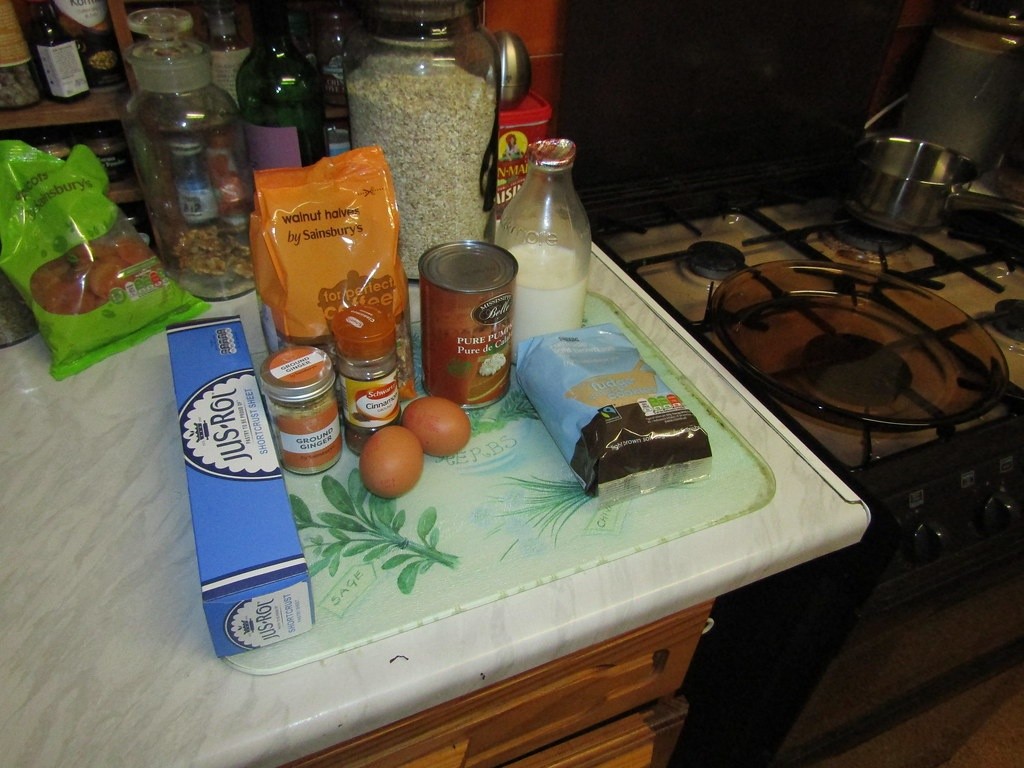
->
[0,0,182,266]
[274,597,715,768]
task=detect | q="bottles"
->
[0,0,155,349]
[332,306,400,455]
[121,1,361,303]
[494,137,588,366]
[260,346,339,476]
[345,0,500,285]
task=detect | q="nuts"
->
[171,223,254,279]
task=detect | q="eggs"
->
[359,426,425,499]
[402,396,470,457]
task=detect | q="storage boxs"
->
[165,314,315,659]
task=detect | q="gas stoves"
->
[509,105,1023,508]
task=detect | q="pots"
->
[844,136,982,238]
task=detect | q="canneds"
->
[418,241,519,407]
[261,306,403,476]
